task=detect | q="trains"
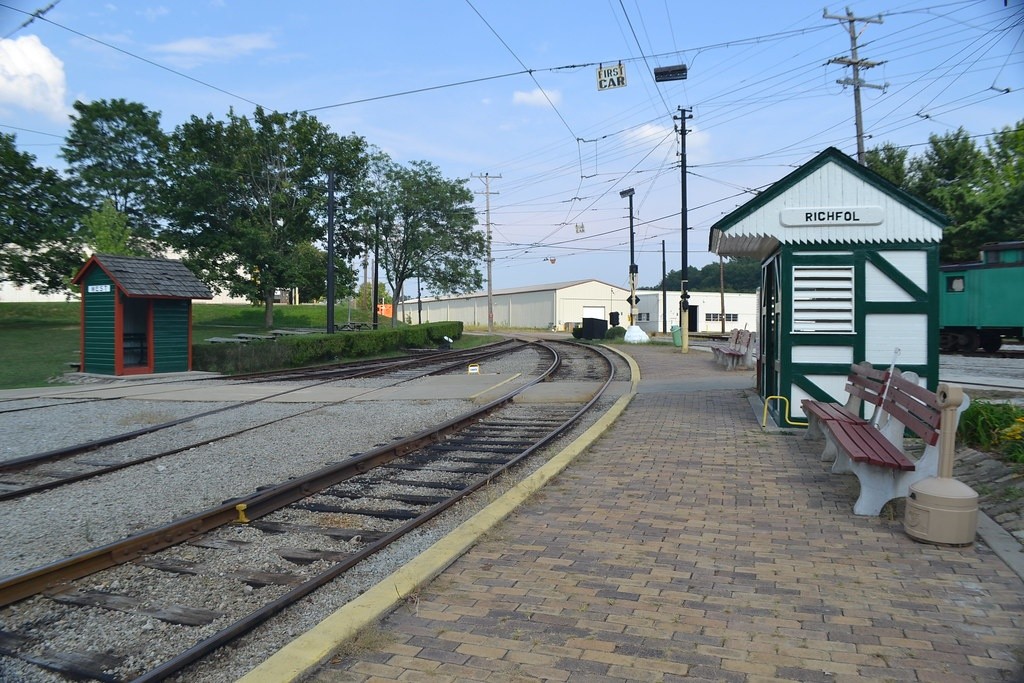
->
[940,239,1024,356]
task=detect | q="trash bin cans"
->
[670,324,682,347]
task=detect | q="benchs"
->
[800,361,901,462]
[711,329,755,370]
[826,372,971,517]
